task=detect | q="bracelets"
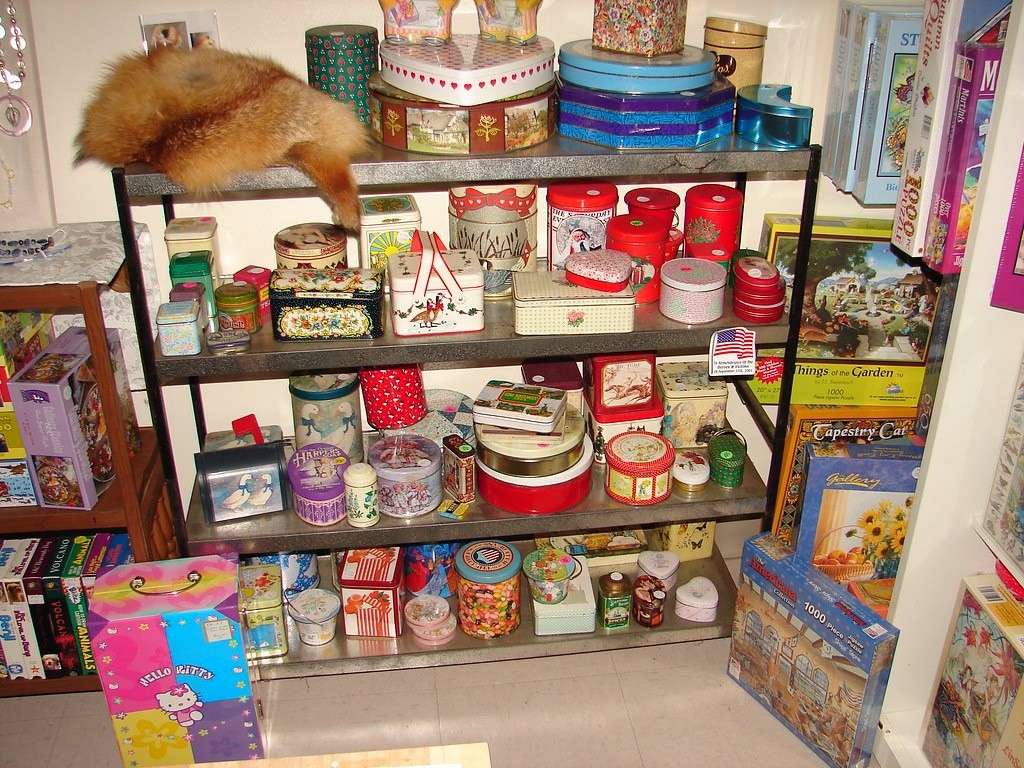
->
[0,237,54,257]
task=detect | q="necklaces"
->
[0,159,16,210]
[1,0,34,137]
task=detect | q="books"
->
[819,0,1013,275]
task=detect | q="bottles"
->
[598,572,632,632]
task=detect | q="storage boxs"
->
[5,326,142,512]
[725,0,1024,768]
[87,548,265,768]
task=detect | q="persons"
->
[151,24,182,49]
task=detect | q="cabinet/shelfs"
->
[0,223,185,696]
[110,146,824,675]
